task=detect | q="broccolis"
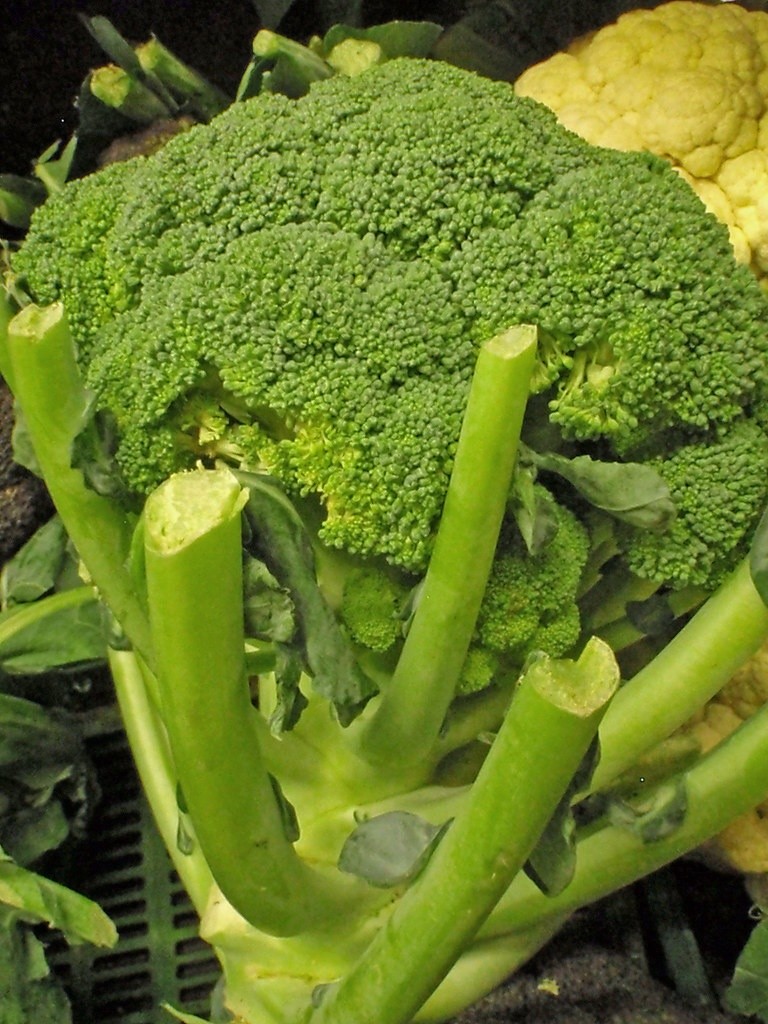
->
[0,0,768,1024]
